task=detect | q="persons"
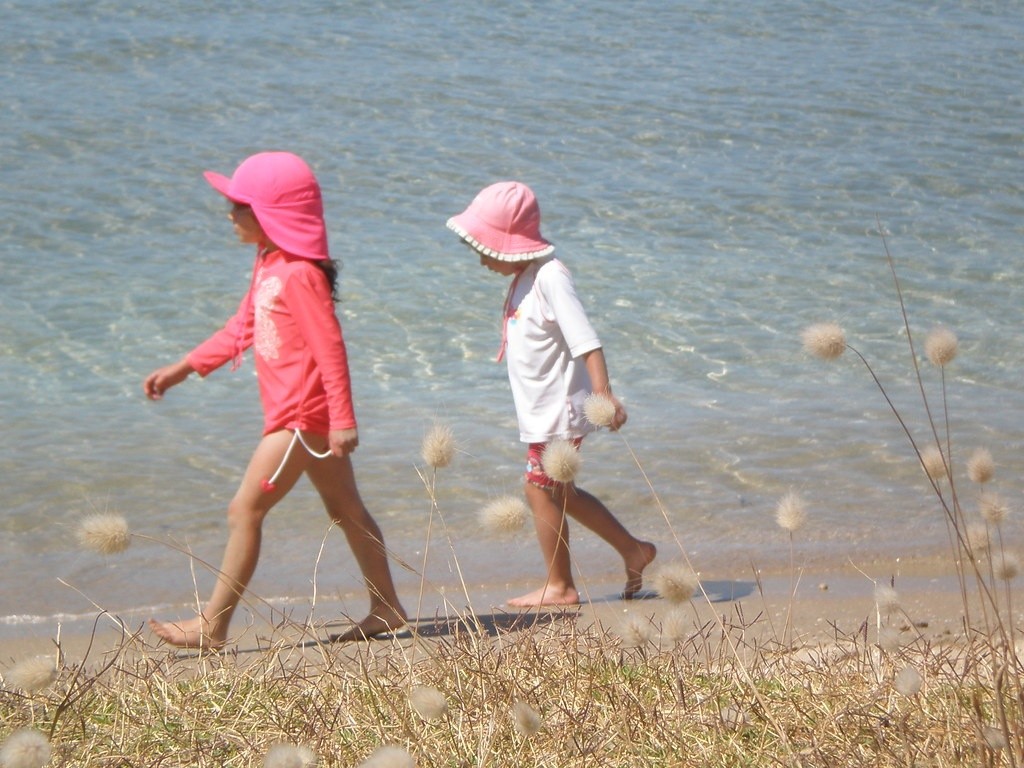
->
[143,151,407,652]
[446,180,657,607]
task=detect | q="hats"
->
[202,152,327,261]
[445,182,555,263]
